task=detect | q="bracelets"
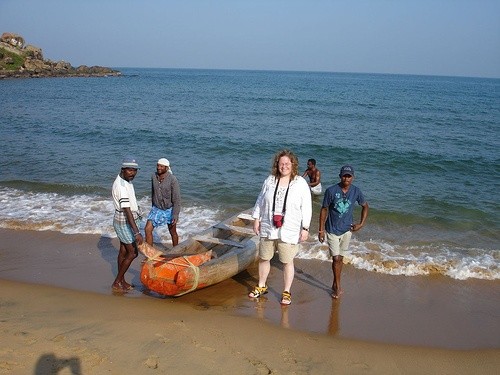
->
[133,232,141,237]
[303,227,309,232]
[318,230,324,233]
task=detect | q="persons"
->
[249,150,312,304]
[302,158,323,193]
[145,158,180,247]
[318,166,368,298]
[112,157,144,291]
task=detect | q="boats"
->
[141,207,260,299]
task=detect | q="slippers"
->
[112,282,123,292]
[121,282,135,291]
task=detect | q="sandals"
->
[249,284,268,298]
[280,290,292,305]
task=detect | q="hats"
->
[157,158,170,167]
[339,166,354,177]
[121,159,140,169]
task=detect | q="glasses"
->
[278,163,292,166]
